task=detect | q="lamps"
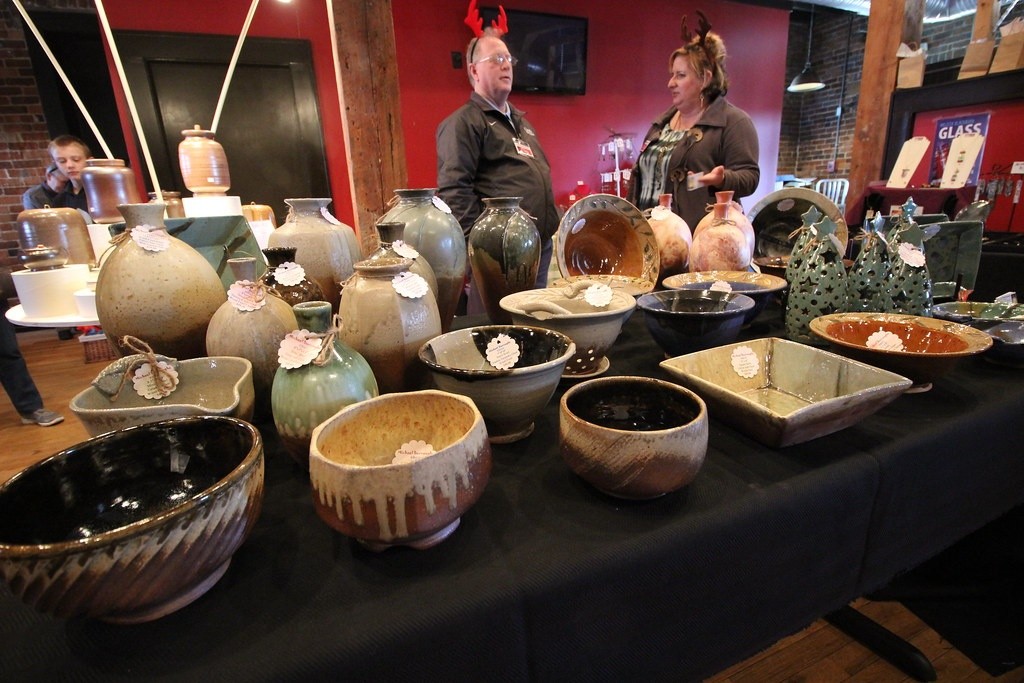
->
[786,5,826,94]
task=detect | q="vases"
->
[96,188,543,464]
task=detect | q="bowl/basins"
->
[308,388,491,553]
[637,271,788,359]
[500,287,637,374]
[70,355,257,438]
[419,325,576,445]
[0,416,266,623]
[809,312,993,393]
[932,301,1024,367]
[559,375,709,500]
[555,192,661,309]
[659,337,912,446]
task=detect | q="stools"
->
[694,593,999,681]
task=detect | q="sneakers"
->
[20,408,64,427]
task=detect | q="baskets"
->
[79,333,117,364]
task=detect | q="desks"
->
[1,336,1024,683]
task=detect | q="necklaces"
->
[654,104,709,183]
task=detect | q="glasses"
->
[475,55,518,66]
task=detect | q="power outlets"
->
[451,52,463,68]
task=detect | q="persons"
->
[0,292,64,426]
[23,163,82,340]
[625,32,760,238]
[47,135,96,212]
[436,37,559,315]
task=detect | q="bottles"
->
[259,188,543,395]
[207,257,298,397]
[96,204,228,360]
[272,301,379,470]
[647,190,755,275]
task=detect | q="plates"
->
[108,215,268,294]
[746,187,848,273]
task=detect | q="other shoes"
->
[58,330,74,340]
